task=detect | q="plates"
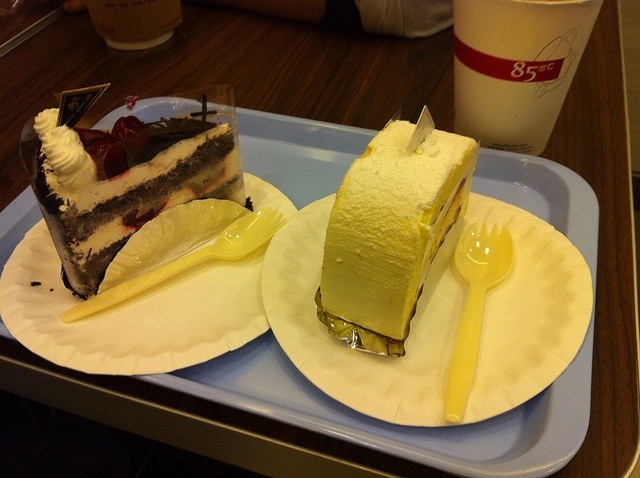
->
[1,172,297,376]
[262,187,594,428]
[1,94,599,473]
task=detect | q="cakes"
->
[315,104,481,358]
[19,82,246,297]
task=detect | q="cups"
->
[454,2,602,158]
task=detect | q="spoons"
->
[445,220,515,426]
[59,206,286,322]
